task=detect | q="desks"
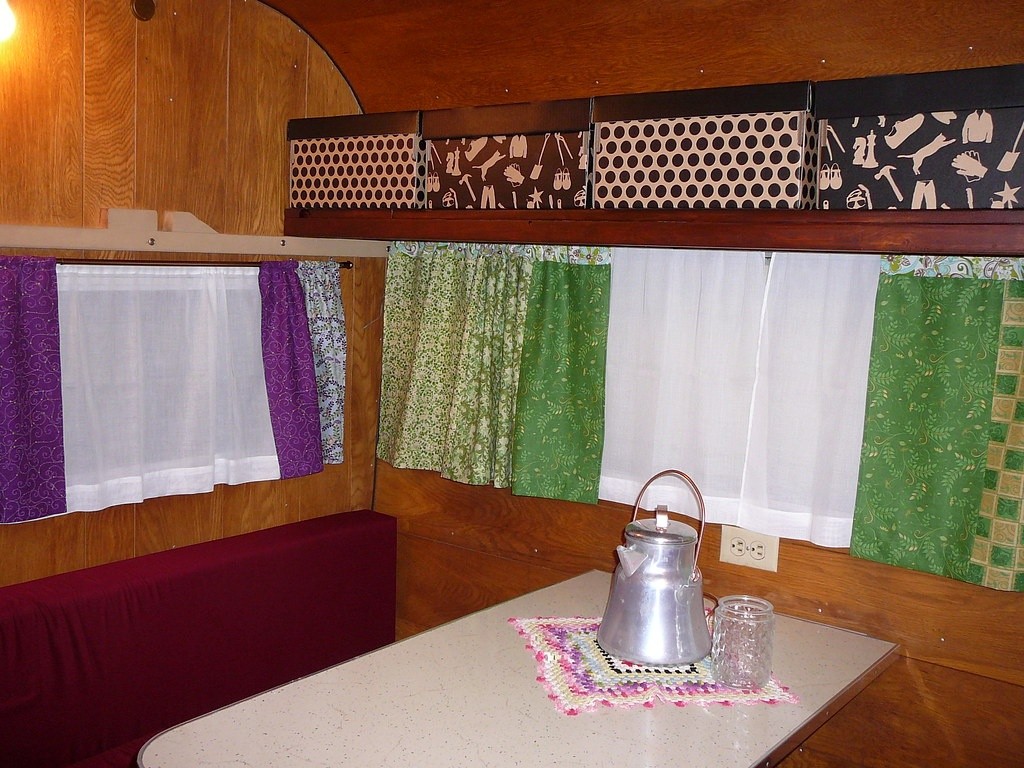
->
[128,565,907,766]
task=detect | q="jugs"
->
[597,467,712,666]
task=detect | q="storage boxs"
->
[422,98,593,209]
[590,78,818,209]
[812,63,1023,211]
[285,108,427,210]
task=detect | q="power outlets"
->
[719,525,780,573]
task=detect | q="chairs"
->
[1,508,398,767]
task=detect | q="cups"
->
[709,595,774,688]
